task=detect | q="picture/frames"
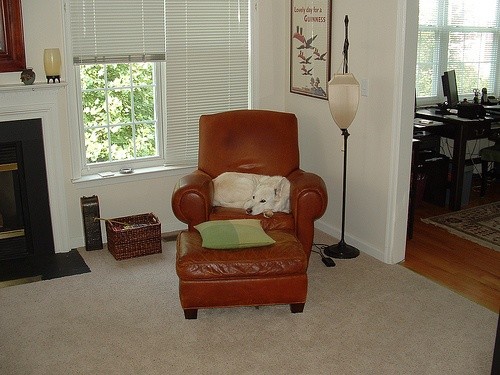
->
[0,0,28,73]
[290,0,332,101]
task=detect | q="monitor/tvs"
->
[441,69,459,108]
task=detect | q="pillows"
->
[193,218,277,250]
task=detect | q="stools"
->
[176,231,308,319]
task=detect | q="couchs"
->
[171,110,328,272]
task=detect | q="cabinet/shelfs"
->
[0,83,70,254]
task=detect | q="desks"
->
[416,104,500,210]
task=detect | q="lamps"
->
[323,14,360,261]
[43,48,62,83]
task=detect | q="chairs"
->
[479,146,500,196]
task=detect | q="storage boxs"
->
[105,212,163,261]
[414,118,446,153]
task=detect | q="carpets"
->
[419,202,500,252]
[0,248,91,288]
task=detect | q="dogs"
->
[210,171,290,219]
